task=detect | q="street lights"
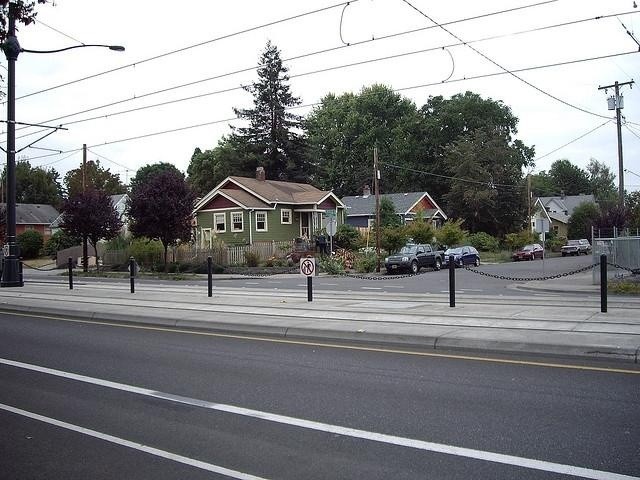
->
[2,34,124,295]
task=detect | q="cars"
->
[443,245,481,267]
[512,243,546,260]
[561,238,591,255]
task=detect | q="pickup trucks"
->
[384,243,445,273]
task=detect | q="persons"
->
[316,232,328,256]
